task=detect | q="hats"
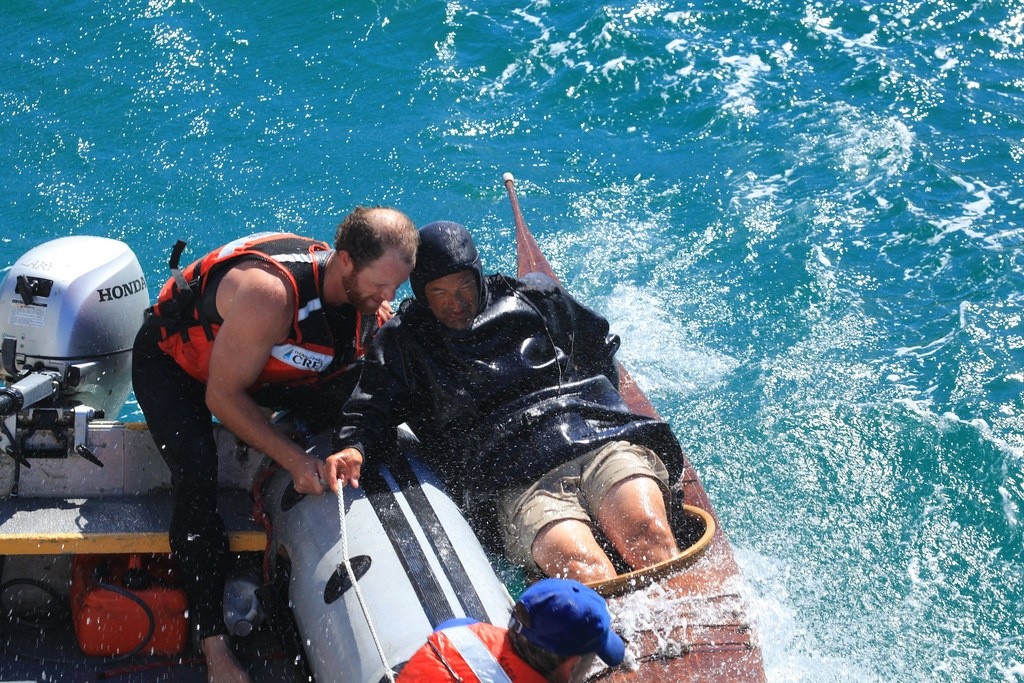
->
[410,221,483,309]
[508,578,625,668]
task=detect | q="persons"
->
[324,221,683,584]
[392,578,625,683]
[132,205,419,682]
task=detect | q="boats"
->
[488,173,766,682]
